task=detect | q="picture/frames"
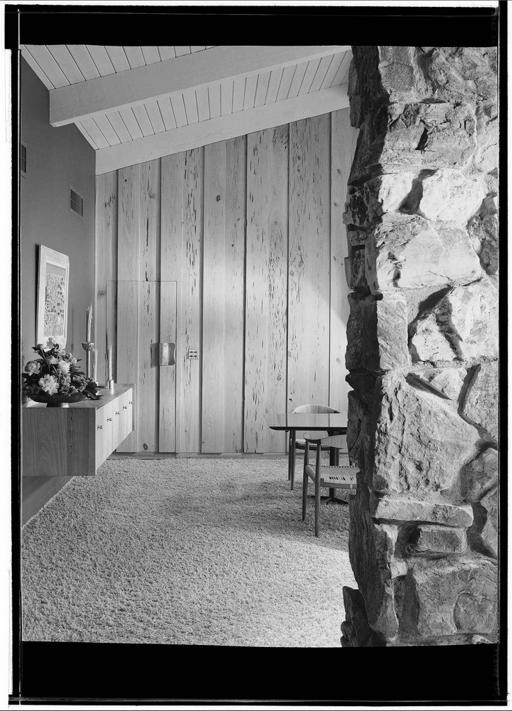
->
[36,244,71,349]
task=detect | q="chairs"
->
[269,403,358,538]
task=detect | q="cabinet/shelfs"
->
[21,385,134,479]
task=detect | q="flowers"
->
[21,348,100,404]
